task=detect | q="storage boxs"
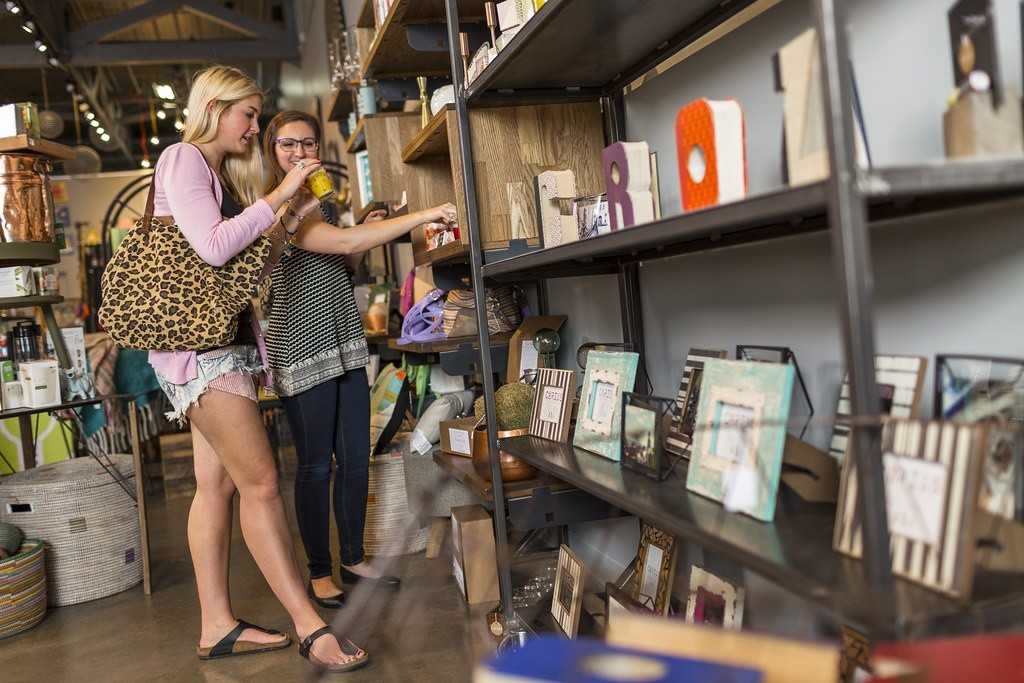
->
[0,328,85,410]
[440,417,482,458]
[486,0,552,64]
[450,503,501,605]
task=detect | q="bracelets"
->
[280,216,298,242]
[272,230,288,245]
[285,207,305,221]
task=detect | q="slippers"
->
[195,616,290,659]
[298,624,369,671]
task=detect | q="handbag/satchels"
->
[98,142,274,352]
[442,280,523,338]
[394,287,533,345]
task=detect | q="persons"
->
[147,65,370,672]
[260,109,457,608]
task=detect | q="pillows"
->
[371,361,410,455]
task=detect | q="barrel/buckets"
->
[0,153,57,243]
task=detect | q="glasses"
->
[274,137,319,152]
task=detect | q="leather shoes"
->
[339,564,400,586]
[306,579,348,609]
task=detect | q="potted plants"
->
[471,380,540,483]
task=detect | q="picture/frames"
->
[522,345,1024,642]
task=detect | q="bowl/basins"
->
[472,426,538,481]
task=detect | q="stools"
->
[403,441,482,559]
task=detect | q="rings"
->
[285,200,292,204]
[300,163,305,168]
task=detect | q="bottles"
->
[359,77,377,114]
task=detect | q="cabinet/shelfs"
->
[324,0,614,586]
[445,0,1024,683]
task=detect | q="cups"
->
[307,167,336,199]
[0,321,44,410]
